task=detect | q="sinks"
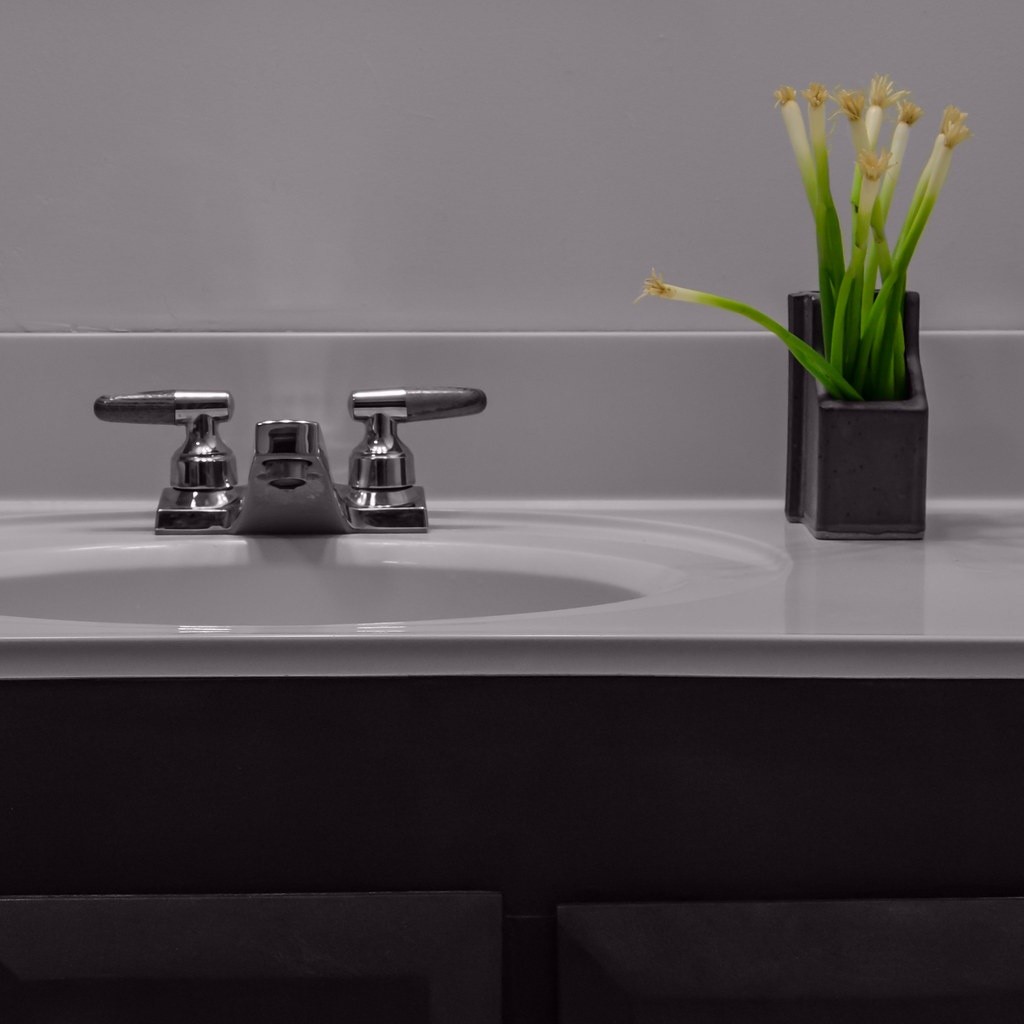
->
[0,497,799,661]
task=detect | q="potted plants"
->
[634,73,971,539]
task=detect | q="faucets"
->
[238,411,349,531]
